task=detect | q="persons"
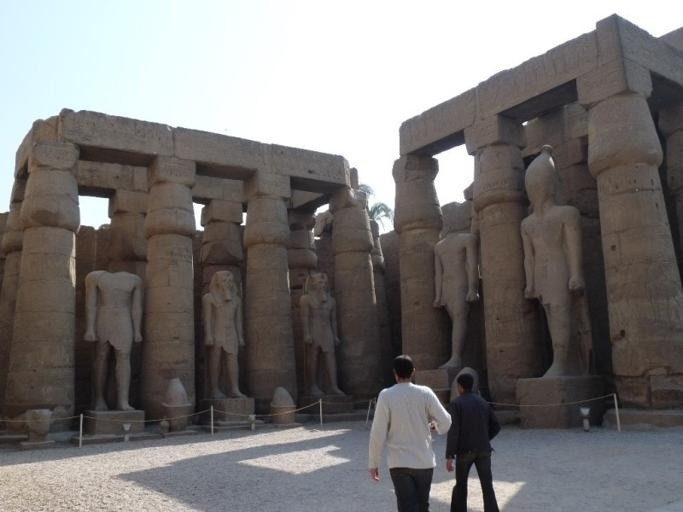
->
[519,144,586,379]
[366,353,451,512]
[444,374,501,512]
[430,201,480,369]
[82,270,143,412]
[200,271,246,399]
[297,272,344,396]
